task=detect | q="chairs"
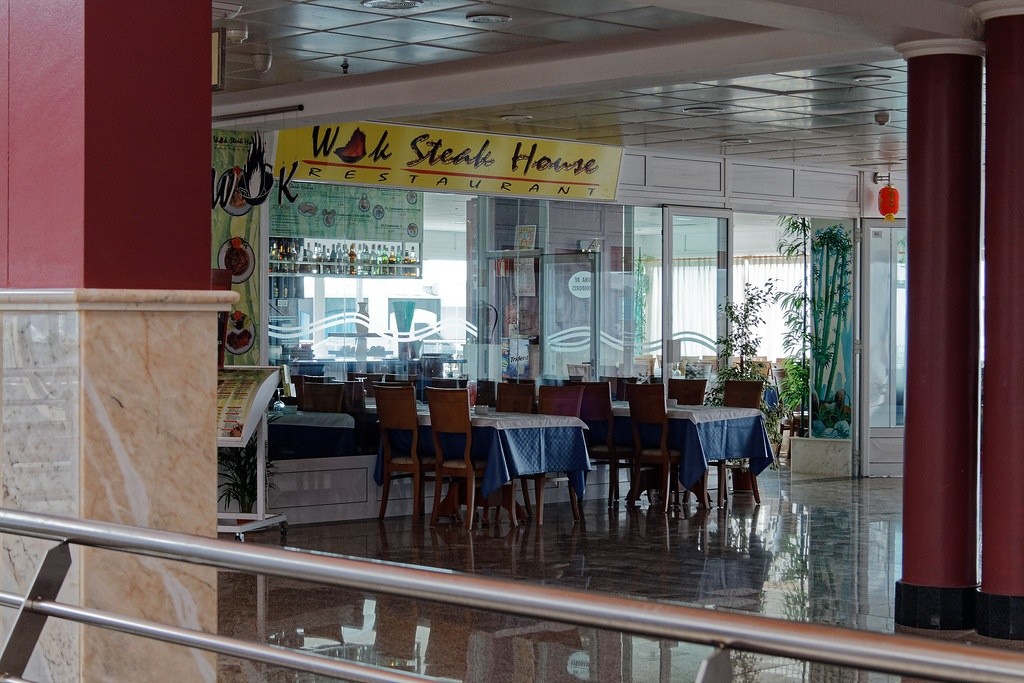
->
[373,383,467,525]
[425,386,522,534]
[627,378,708,511]
[578,380,657,511]
[682,378,769,511]
[619,381,711,516]
[494,381,586,528]
[568,352,811,410]
[280,353,469,415]
[774,376,811,459]
[451,382,536,526]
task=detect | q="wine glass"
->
[274,388,285,411]
[675,362,681,378]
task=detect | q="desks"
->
[583,399,774,511]
[370,405,588,527]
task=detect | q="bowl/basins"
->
[488,407,496,415]
[474,404,489,415]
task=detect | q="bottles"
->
[284,281,288,297]
[273,280,279,298]
[270,239,418,277]
